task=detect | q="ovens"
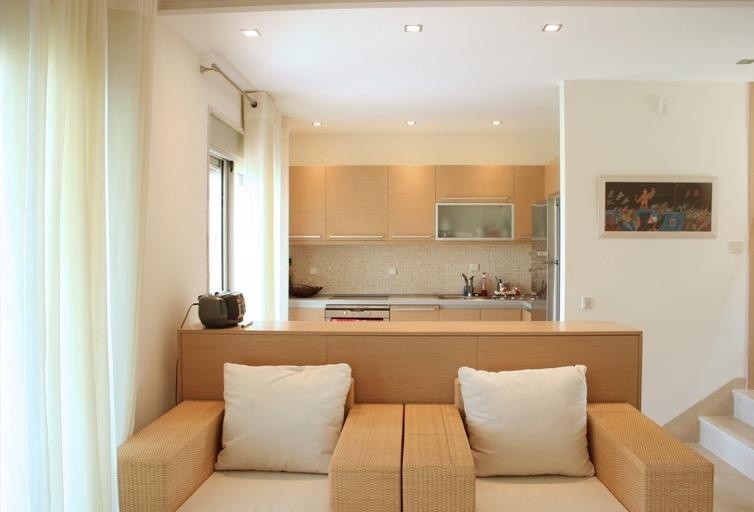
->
[323,305,390,320]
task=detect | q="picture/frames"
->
[594,171,722,244]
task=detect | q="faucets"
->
[461,273,475,297]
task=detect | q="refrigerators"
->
[543,194,561,321]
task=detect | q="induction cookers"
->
[327,294,390,300]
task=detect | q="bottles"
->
[480,272,488,296]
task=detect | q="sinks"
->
[437,294,492,299]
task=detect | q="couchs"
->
[406,375,718,510]
[106,364,404,511]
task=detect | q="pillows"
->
[212,355,352,478]
[456,362,598,479]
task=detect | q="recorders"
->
[199,292,246,328]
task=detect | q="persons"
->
[613,188,711,231]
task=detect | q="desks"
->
[177,319,643,412]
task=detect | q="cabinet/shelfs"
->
[388,162,436,243]
[288,307,323,320]
[390,305,439,320]
[437,162,545,242]
[521,310,545,322]
[289,164,389,246]
[439,309,521,320]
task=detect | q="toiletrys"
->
[481,272,487,296]
